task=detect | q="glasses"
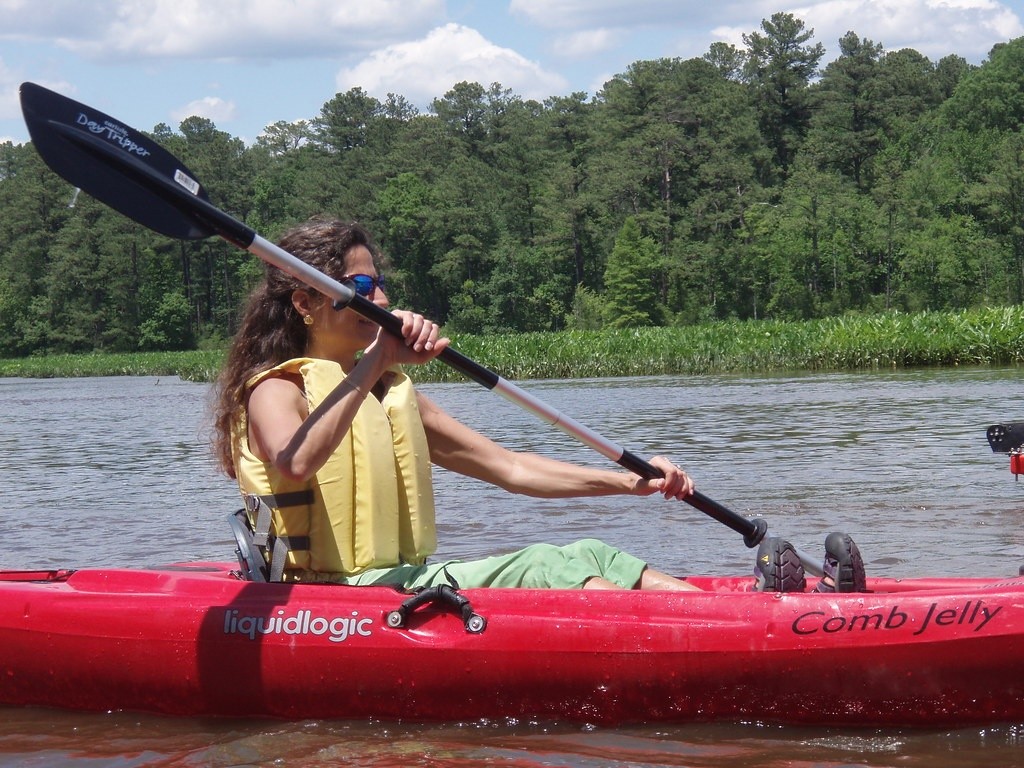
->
[309,274,385,296]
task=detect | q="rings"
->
[674,464,682,470]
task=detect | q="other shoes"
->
[750,539,806,594]
[811,532,867,594]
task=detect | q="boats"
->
[1,554,1024,738]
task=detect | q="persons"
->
[203,223,863,598]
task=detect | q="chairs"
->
[227,507,273,584]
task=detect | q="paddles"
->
[19,82,828,578]
